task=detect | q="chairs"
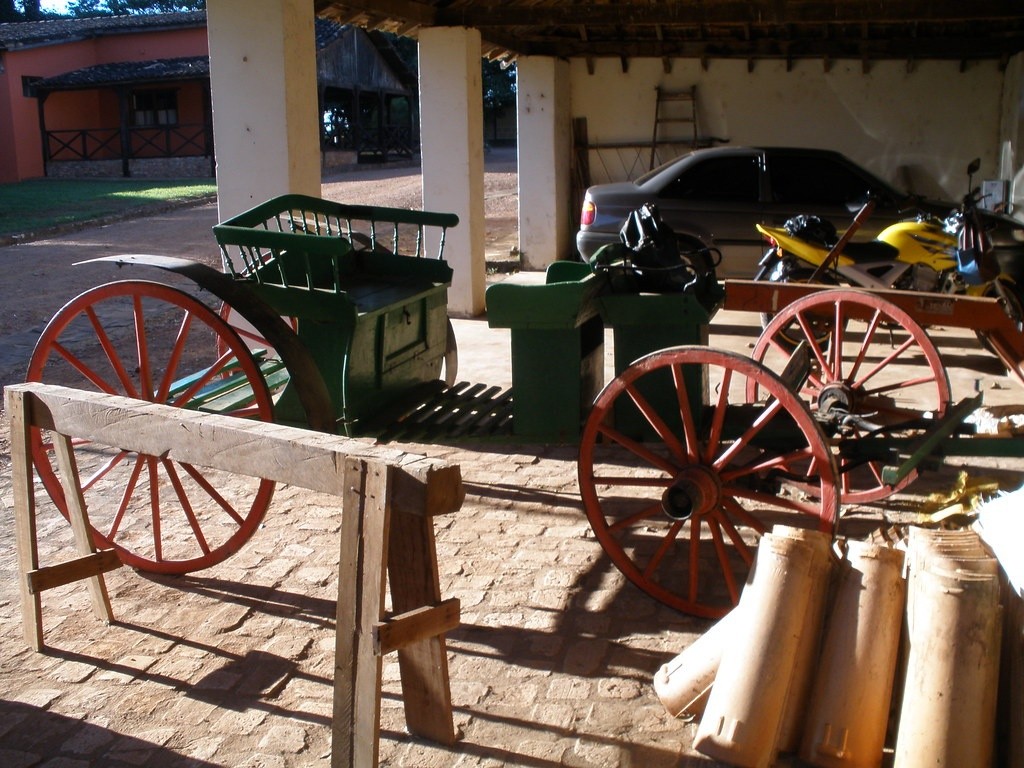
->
[484,261,724,442]
[213,193,458,436]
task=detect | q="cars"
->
[575,144,1024,292]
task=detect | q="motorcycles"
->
[751,157,1024,356]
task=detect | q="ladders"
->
[648,85,698,172]
